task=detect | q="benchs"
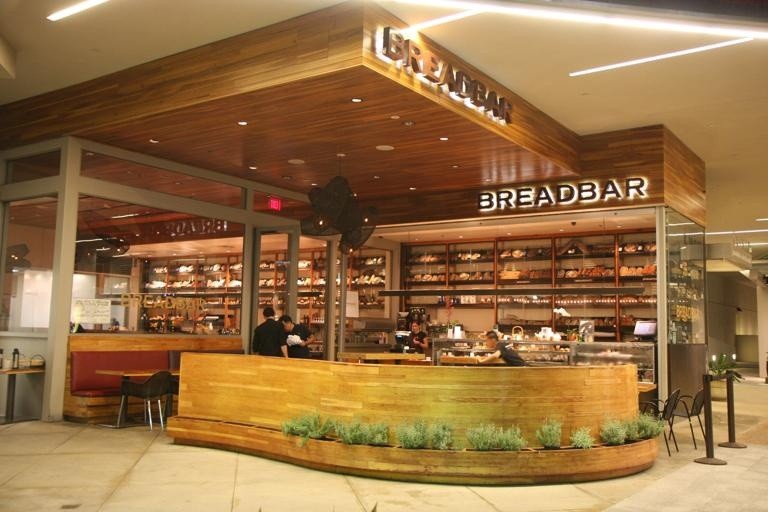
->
[62,336,242,424]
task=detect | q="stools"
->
[116,371,171,430]
[164,375,179,421]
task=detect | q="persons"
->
[279,315,316,358]
[252,307,288,358]
[477,330,527,366]
[408,321,428,354]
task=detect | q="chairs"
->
[669,388,706,449]
[639,388,680,456]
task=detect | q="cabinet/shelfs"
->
[427,338,656,384]
[400,227,657,309]
[145,256,385,309]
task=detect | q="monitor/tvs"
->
[395,331,413,345]
[630,320,657,342]
